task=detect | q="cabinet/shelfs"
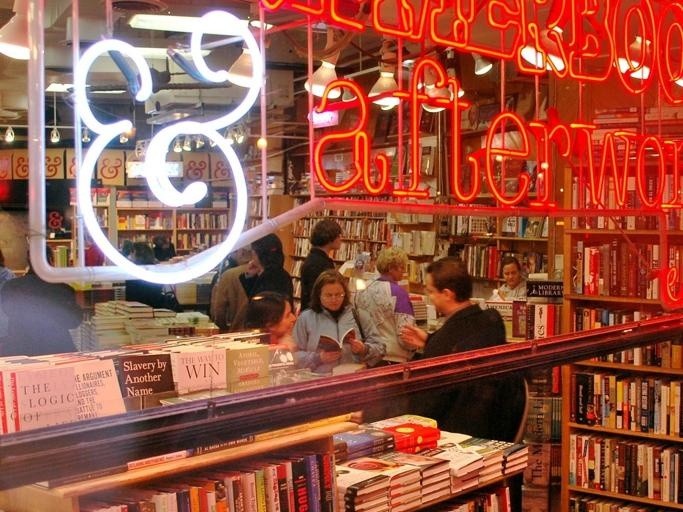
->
[292,123,681,512]
[73,188,291,281]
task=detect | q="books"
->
[0,328,528,511]
[69,187,230,269]
[291,177,683,512]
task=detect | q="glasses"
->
[321,292,346,300]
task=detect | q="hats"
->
[252,234,284,275]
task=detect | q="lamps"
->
[303,14,494,113]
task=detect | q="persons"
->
[125,217,528,511]
